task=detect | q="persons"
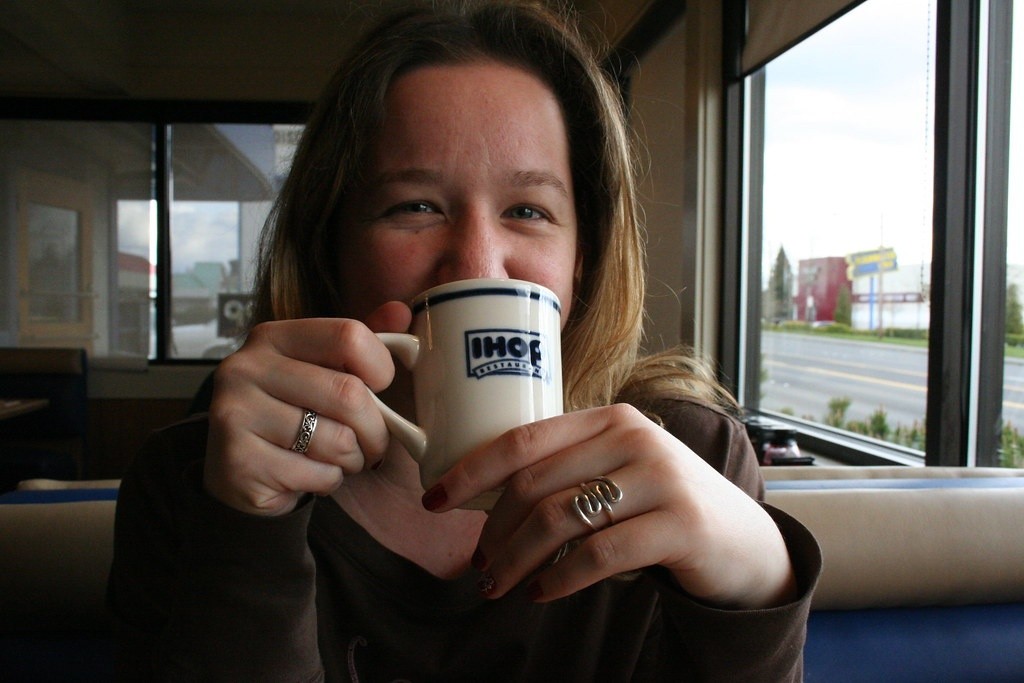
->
[102,0,824,683]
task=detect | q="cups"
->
[362,279,565,510]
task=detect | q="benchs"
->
[0,468,1024,683]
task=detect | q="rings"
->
[291,408,318,454]
[572,475,623,531]
